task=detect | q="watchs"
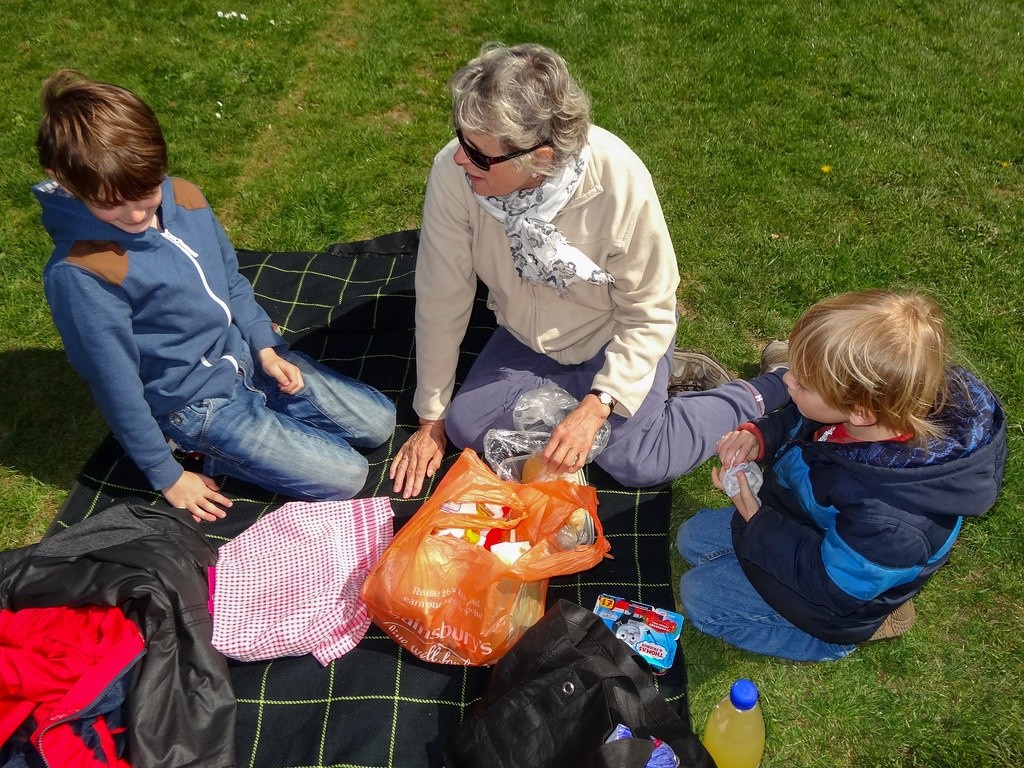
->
[587,389,614,419]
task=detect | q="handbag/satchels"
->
[204,496,393,665]
[431,599,719,768]
[359,447,611,665]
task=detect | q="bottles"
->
[703,678,766,767]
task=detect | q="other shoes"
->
[863,596,916,643]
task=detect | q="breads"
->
[522,451,588,536]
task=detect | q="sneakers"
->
[757,342,793,374]
[666,350,731,397]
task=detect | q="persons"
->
[34,69,397,524]
[677,291,1006,661]
[389,44,791,498]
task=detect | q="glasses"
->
[455,128,552,170]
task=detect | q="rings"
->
[402,458,409,461]
